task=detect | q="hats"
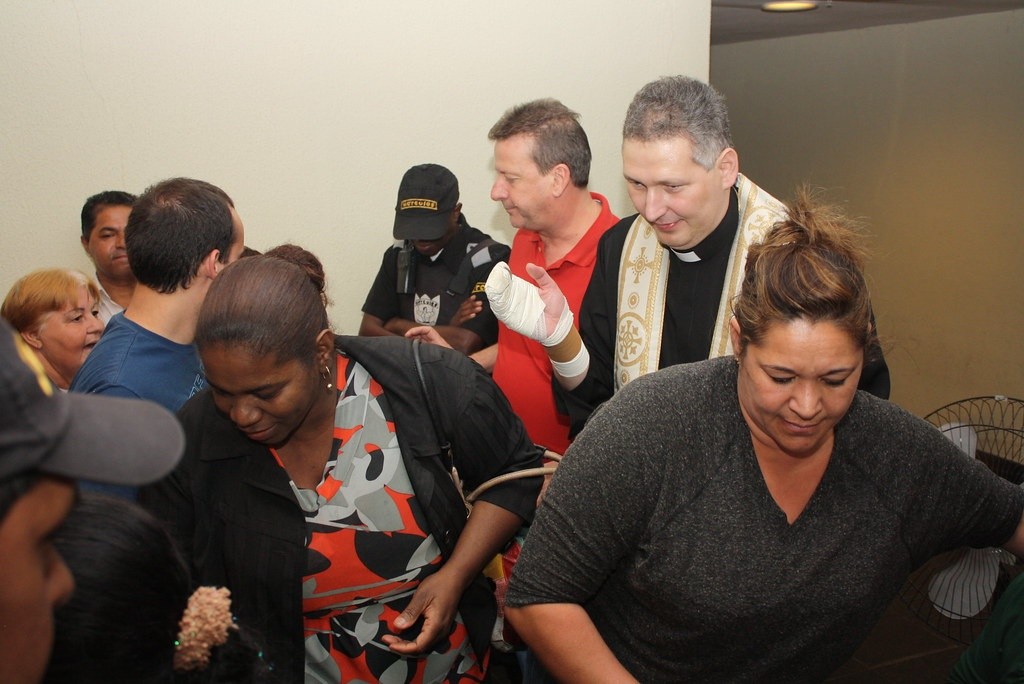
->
[393,164,460,240]
[0,317,187,484]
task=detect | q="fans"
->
[899,396,1024,647]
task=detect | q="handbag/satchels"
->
[413,337,532,652]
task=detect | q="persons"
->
[0,269,105,394]
[404,97,622,585]
[264,245,325,294]
[502,184,1023,684]
[137,242,546,684]
[69,177,244,500]
[485,74,891,443]
[359,163,513,354]
[78,192,136,330]
[0,317,186,684]
[51,495,272,684]
